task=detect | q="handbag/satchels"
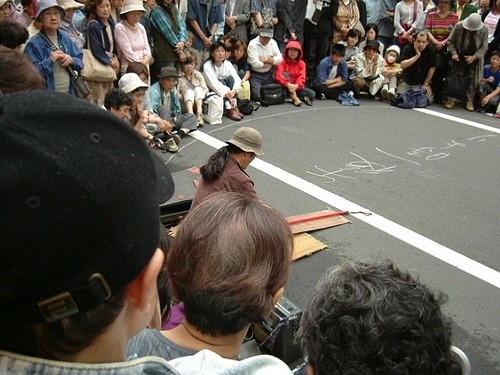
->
[81,47,118,83]
[260,84,285,105]
[250,6,273,35]
[71,76,91,99]
[237,98,260,114]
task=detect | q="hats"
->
[363,40,381,54]
[118,72,149,95]
[157,66,180,79]
[259,27,275,37]
[120,0,146,18]
[3,88,175,327]
[31,1,85,22]
[462,13,484,30]
[224,126,265,158]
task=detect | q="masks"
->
[387,55,396,63]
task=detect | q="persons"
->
[0,21,30,52]
[247,23,283,99]
[185,0,362,75]
[177,48,209,125]
[203,33,251,121]
[297,258,452,375]
[273,39,315,107]
[358,0,500,119]
[143,66,197,152]
[0,90,181,375]
[350,40,385,99]
[0,45,47,93]
[311,44,354,100]
[126,190,294,375]
[185,30,195,48]
[100,88,133,120]
[0,0,84,97]
[119,72,164,149]
[73,0,187,109]
[190,127,265,209]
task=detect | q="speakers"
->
[254,294,304,364]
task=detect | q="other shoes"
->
[227,106,244,120]
[293,96,314,108]
[197,114,205,126]
[467,100,476,111]
[445,98,457,109]
[156,127,181,153]
[381,86,395,101]
